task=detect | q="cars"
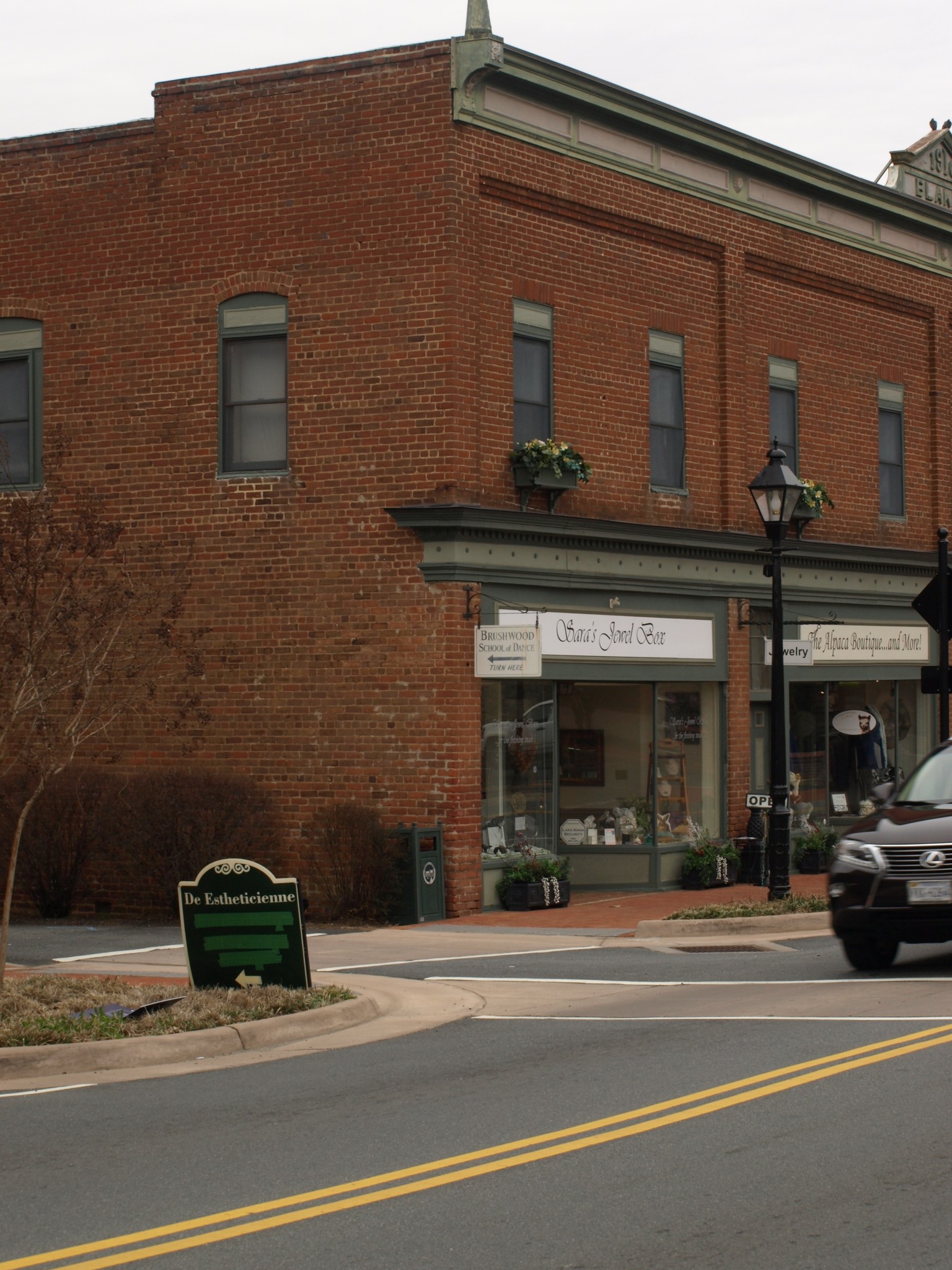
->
[820,737,951,969]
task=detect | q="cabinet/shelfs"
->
[646,739,693,843]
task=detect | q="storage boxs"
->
[582,828,598,845]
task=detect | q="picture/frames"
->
[560,728,607,788]
[830,791,851,815]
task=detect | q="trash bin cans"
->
[370,820,447,926]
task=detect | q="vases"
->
[512,463,581,490]
[792,500,823,520]
[689,864,725,889]
[798,850,829,875]
[504,881,571,912]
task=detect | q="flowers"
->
[508,436,593,487]
[681,815,741,888]
[495,832,573,903]
[791,815,841,865]
[795,477,835,519]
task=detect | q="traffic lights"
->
[744,440,808,899]
